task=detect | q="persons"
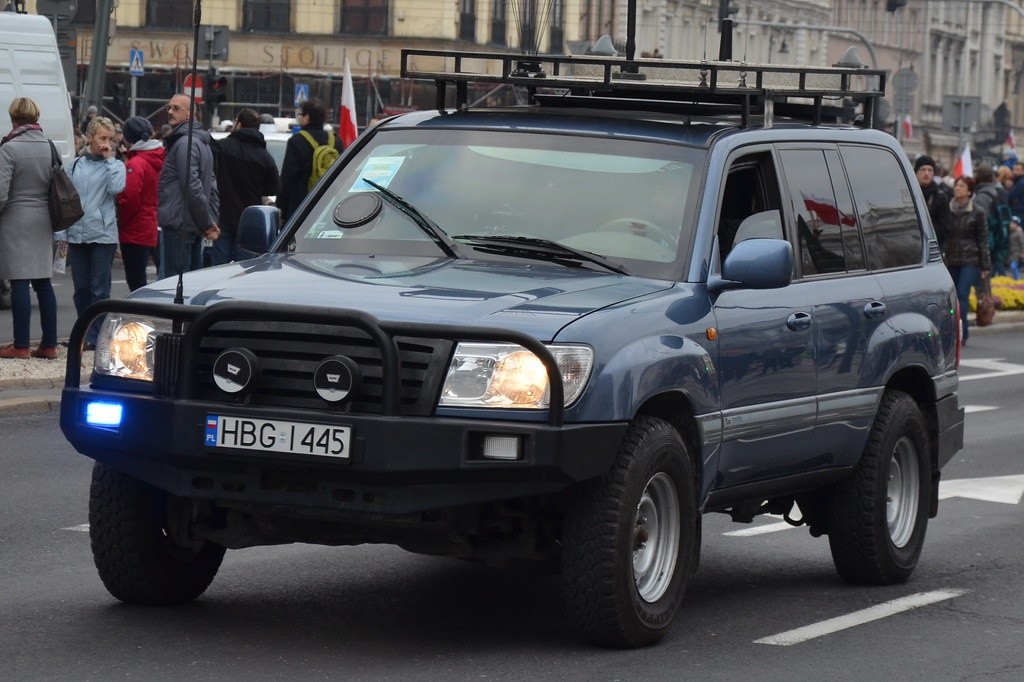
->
[913,151,1024,346]
[798,215,921,275]
[0,93,387,358]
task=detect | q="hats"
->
[122,116,153,143]
[259,114,274,124]
[914,156,936,174]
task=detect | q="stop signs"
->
[182,73,204,103]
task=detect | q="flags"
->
[340,61,359,150]
[802,188,855,228]
[905,114,1016,177]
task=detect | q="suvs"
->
[58,48,967,647]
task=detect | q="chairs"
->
[717,174,763,248]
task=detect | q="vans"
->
[0,11,77,309]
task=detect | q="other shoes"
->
[30,347,58,359]
[63,340,96,351]
[0,346,30,359]
[114,250,122,258]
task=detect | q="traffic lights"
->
[209,72,227,111]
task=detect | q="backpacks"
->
[975,187,1012,250]
[298,130,339,193]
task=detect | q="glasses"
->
[233,119,237,124]
[165,105,190,112]
[297,113,303,119]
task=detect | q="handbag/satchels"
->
[48,139,84,232]
[976,273,995,327]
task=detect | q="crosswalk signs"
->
[295,83,308,108]
[130,50,145,75]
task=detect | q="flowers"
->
[967,276,1023,314]
